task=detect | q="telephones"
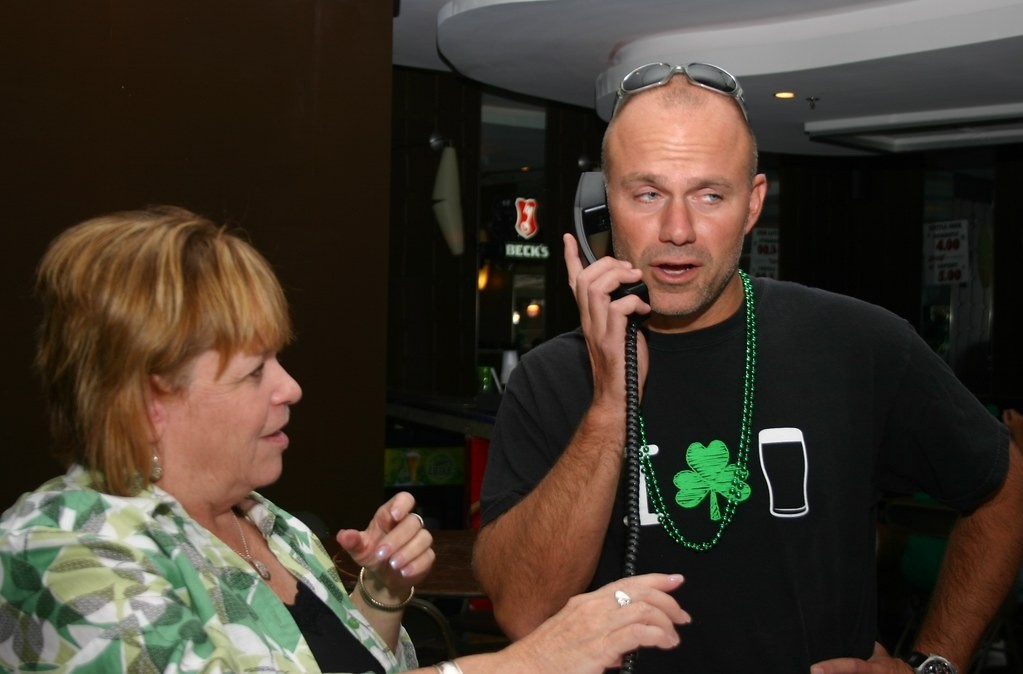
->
[573,170,651,317]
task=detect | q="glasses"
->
[612,62,749,123]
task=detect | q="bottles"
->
[476,366,493,394]
[758,427,810,518]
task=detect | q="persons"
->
[0,205,690,674]
[469,73,1023,674]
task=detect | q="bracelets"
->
[358,568,415,610]
[433,659,463,674]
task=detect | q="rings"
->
[614,591,630,607]
[412,513,424,527]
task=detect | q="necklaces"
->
[228,511,271,580]
[640,270,758,552]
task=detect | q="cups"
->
[502,351,519,385]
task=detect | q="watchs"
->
[906,653,957,674]
